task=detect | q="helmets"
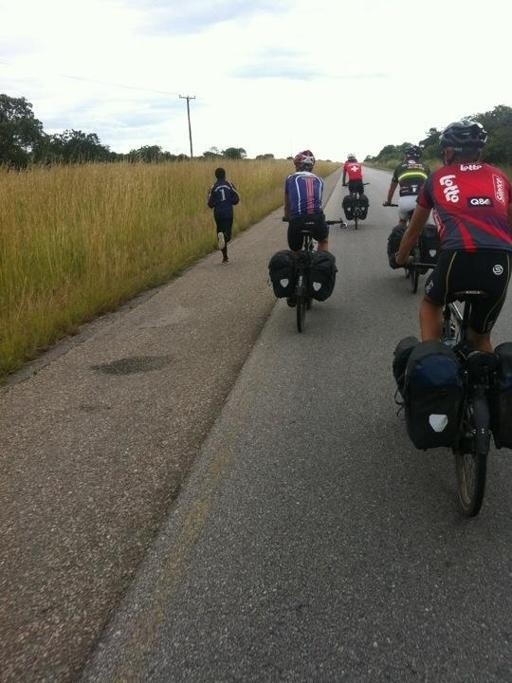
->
[347,153,356,161]
[405,145,422,158]
[441,121,488,147]
[294,150,315,169]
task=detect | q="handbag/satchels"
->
[268,249,299,298]
[343,195,354,220]
[420,224,441,268]
[387,224,414,269]
[307,249,337,301]
[358,194,368,219]
[494,342,512,450]
[392,335,463,450]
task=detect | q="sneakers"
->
[217,232,225,249]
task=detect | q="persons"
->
[342,154,364,196]
[284,150,329,306]
[394,121,512,353]
[208,168,239,264]
[387,145,430,224]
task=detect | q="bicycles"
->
[392,257,491,516]
[383,196,427,295]
[281,213,347,334]
[340,180,370,233]
